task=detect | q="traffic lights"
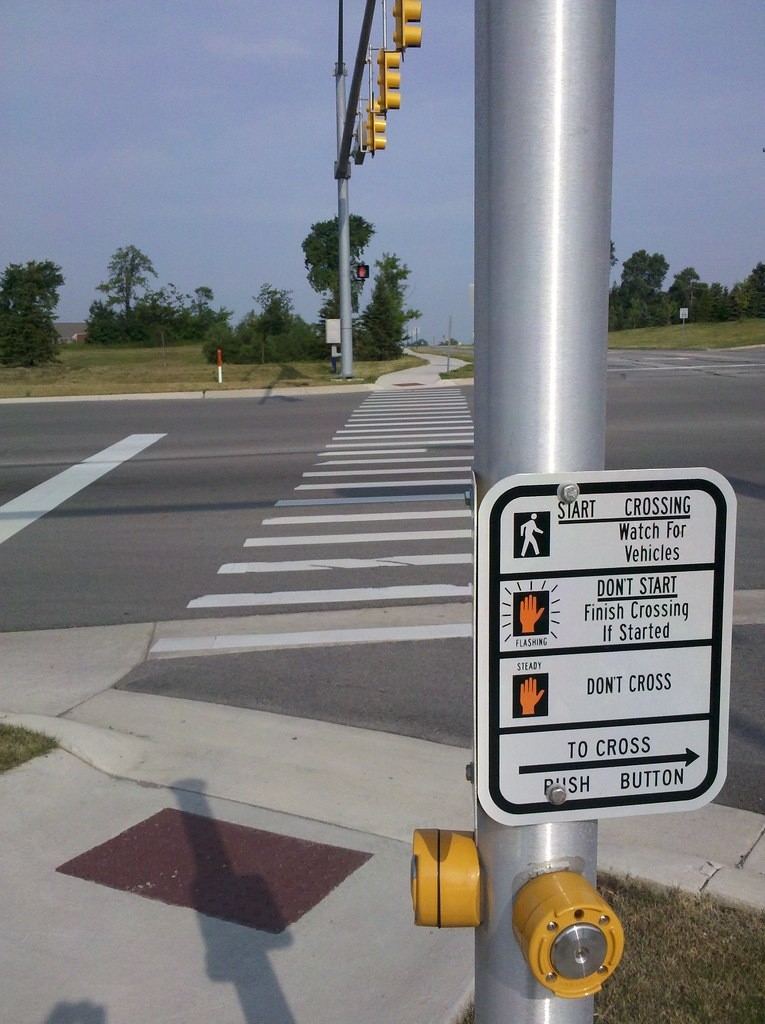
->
[392,0,422,63]
[357,265,369,283]
[366,98,386,158]
[376,48,401,120]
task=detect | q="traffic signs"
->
[476,467,737,828]
